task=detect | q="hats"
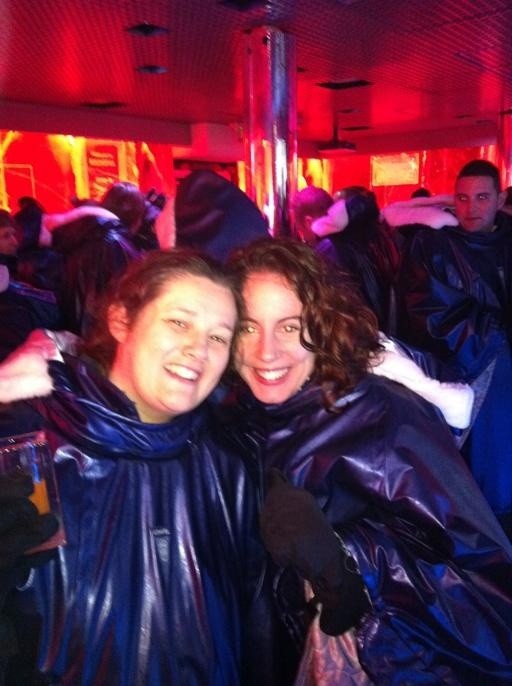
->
[41,206,128,254]
[308,193,382,240]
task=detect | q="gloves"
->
[255,461,374,638]
[0,468,62,593]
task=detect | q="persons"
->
[0,158,509,685]
